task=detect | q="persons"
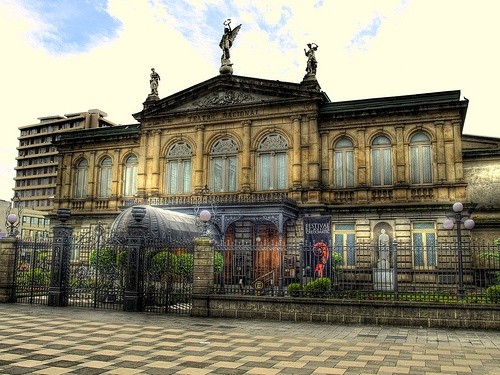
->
[303,42,319,74]
[150,67,161,97]
[222,20,232,60]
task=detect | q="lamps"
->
[5,195,24,237]
[194,200,216,238]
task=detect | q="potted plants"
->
[88,244,225,305]
